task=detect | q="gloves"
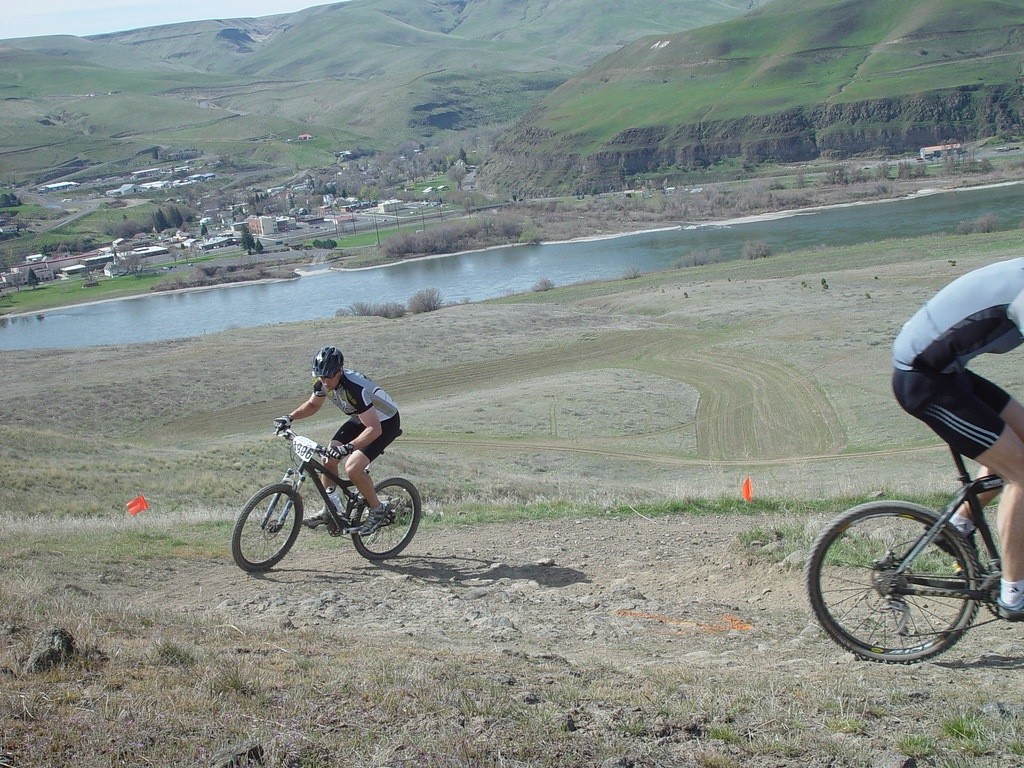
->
[274,414,293,437]
[326,443,354,460]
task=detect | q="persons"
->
[890,258,1024,620]
[273,346,400,535]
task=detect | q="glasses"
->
[319,369,340,379]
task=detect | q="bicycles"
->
[799,441,1024,665]
[230,427,423,572]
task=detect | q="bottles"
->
[326,486,345,514]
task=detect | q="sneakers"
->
[924,524,977,557]
[997,594,1024,622]
[302,508,332,529]
[360,503,392,536]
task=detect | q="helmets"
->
[312,346,344,377]
